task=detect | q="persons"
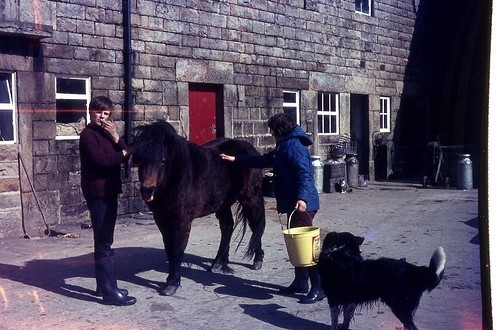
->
[217,112,326,304]
[78,95,137,306]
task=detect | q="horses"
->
[129,119,266,296]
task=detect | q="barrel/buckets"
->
[457,153,474,190]
[310,153,358,194]
[282,208,320,267]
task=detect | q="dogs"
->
[315,231,448,330]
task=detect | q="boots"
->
[280,266,309,295]
[300,265,326,304]
[93,249,136,306]
